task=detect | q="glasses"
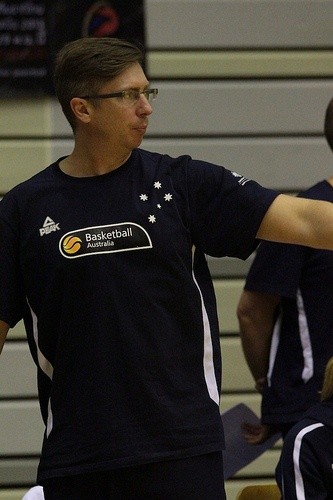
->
[85,88,159,105]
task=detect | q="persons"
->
[0,38,333,500]
[237,97,333,445]
[275,355,333,500]
